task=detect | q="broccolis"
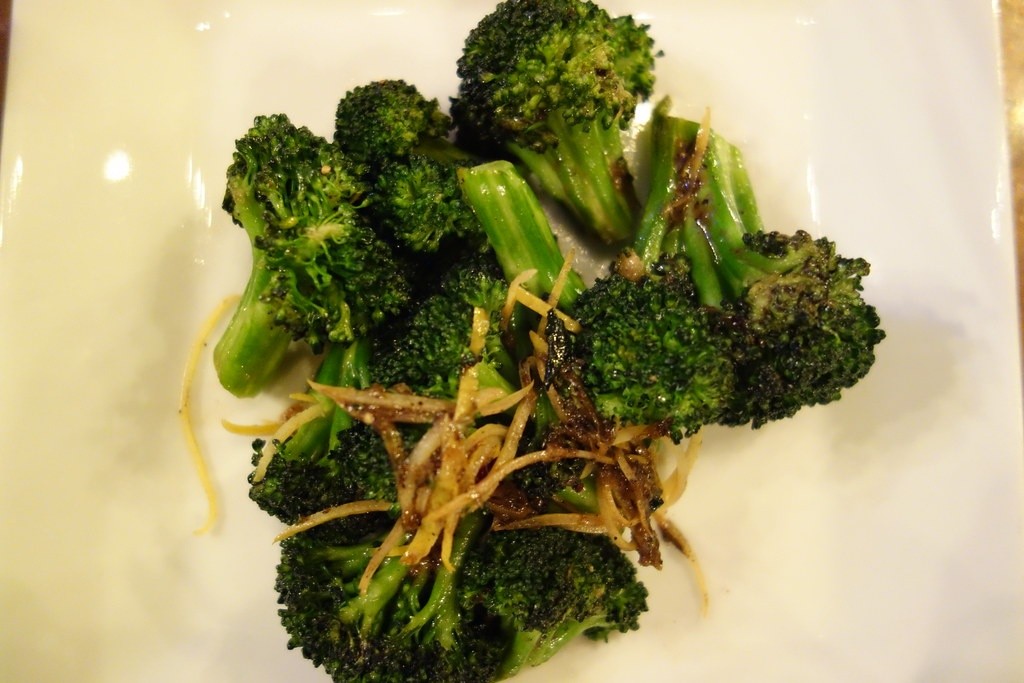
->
[213,0,885,683]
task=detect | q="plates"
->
[1,0,1024,683]
